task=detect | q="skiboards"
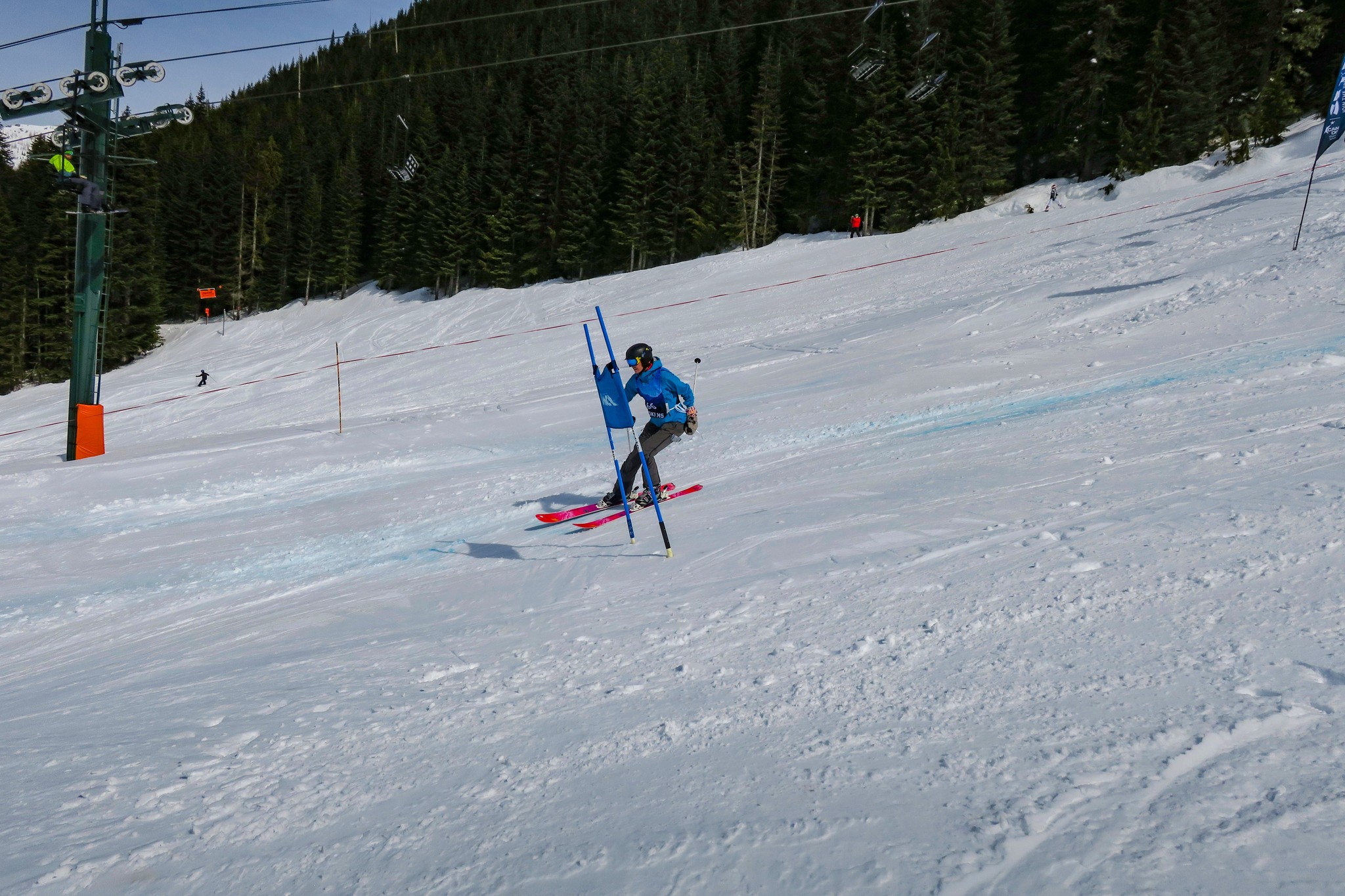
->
[534,483,704,529]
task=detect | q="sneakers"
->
[635,483,661,506]
[603,492,631,504]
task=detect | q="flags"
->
[1316,56,1345,160]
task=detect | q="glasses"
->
[626,356,641,367]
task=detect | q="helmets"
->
[624,343,653,367]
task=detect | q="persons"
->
[598,343,698,511]
[1045,184,1066,212]
[196,370,210,386]
[850,214,861,238]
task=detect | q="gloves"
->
[684,416,698,435]
